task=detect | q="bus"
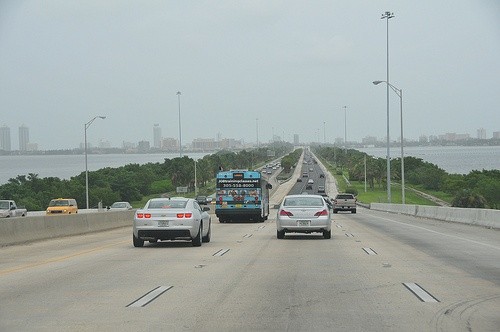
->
[216,169,272,223]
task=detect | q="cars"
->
[262,151,317,177]
[195,196,207,205]
[46,197,78,214]
[107,202,133,210]
[297,178,302,181]
[308,179,313,184]
[306,185,312,189]
[330,193,357,214]
[133,197,212,247]
[318,186,325,192]
[320,173,324,178]
[274,195,334,239]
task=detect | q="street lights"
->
[381,11,395,202]
[256,117,326,146]
[372,81,405,204]
[85,115,106,209]
[176,92,182,158]
[343,105,349,153]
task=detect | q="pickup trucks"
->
[0,200,28,218]
